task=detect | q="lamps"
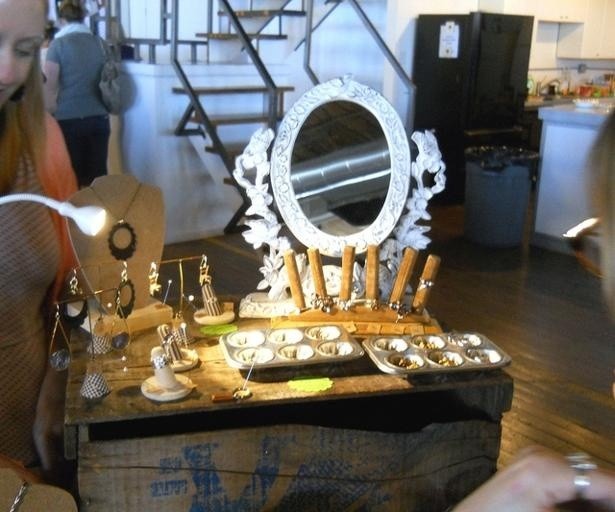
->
[0,192,106,236]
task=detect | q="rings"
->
[153,332,176,370]
[567,450,596,499]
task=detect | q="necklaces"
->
[90,181,145,320]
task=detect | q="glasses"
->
[563,216,603,279]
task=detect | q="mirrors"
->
[232,77,447,319]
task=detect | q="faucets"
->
[536,74,561,96]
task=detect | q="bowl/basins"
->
[572,99,599,109]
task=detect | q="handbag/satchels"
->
[99,60,138,117]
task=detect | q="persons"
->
[448,106,615,511]
[43,0,113,192]
[0,0,80,512]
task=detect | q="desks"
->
[66,332,514,511]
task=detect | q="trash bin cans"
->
[464,145,539,248]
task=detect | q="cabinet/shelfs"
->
[479,0,615,59]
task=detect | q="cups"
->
[579,85,610,97]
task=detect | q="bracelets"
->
[9,480,30,512]
